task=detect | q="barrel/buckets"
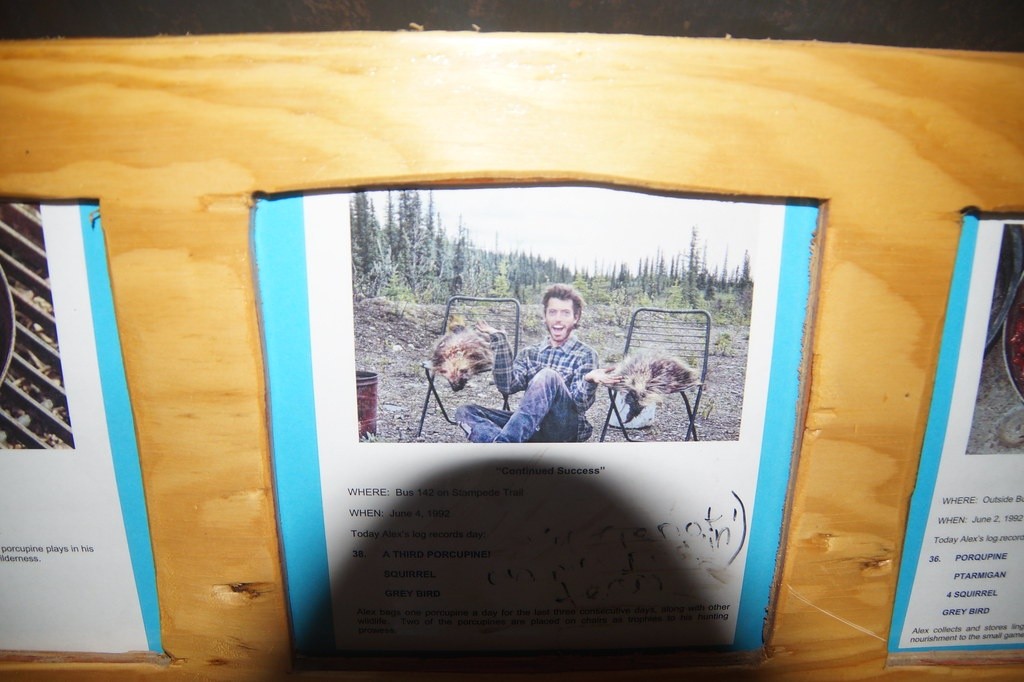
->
[356,370,376,439]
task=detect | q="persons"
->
[455,284,626,445]
